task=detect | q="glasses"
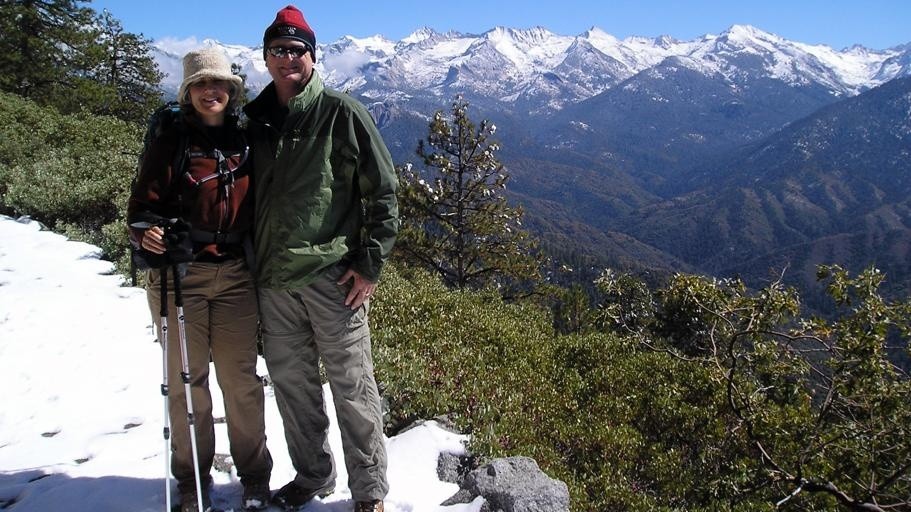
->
[265,47,309,58]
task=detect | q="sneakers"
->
[175,480,213,512]
[353,499,385,512]
[239,471,336,511]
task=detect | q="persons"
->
[126,47,275,512]
[241,3,401,512]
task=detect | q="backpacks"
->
[141,102,251,242]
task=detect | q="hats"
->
[264,5,316,63]
[178,48,242,105]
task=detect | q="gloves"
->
[139,218,192,269]
